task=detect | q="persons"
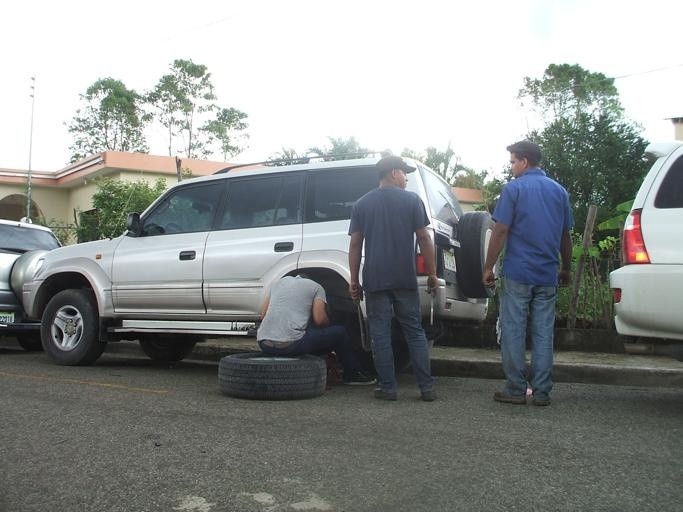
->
[482,139,574,406]
[348,157,440,402]
[256,272,377,386]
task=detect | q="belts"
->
[262,338,293,349]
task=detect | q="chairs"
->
[219,195,255,229]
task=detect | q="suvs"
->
[16,148,504,366]
[607,140,680,352]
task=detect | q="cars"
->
[0,219,64,352]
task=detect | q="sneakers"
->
[494,391,529,405]
[533,396,551,407]
[375,390,399,402]
[342,367,378,386]
[421,388,438,400]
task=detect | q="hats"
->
[376,155,416,175]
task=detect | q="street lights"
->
[22,74,34,225]
